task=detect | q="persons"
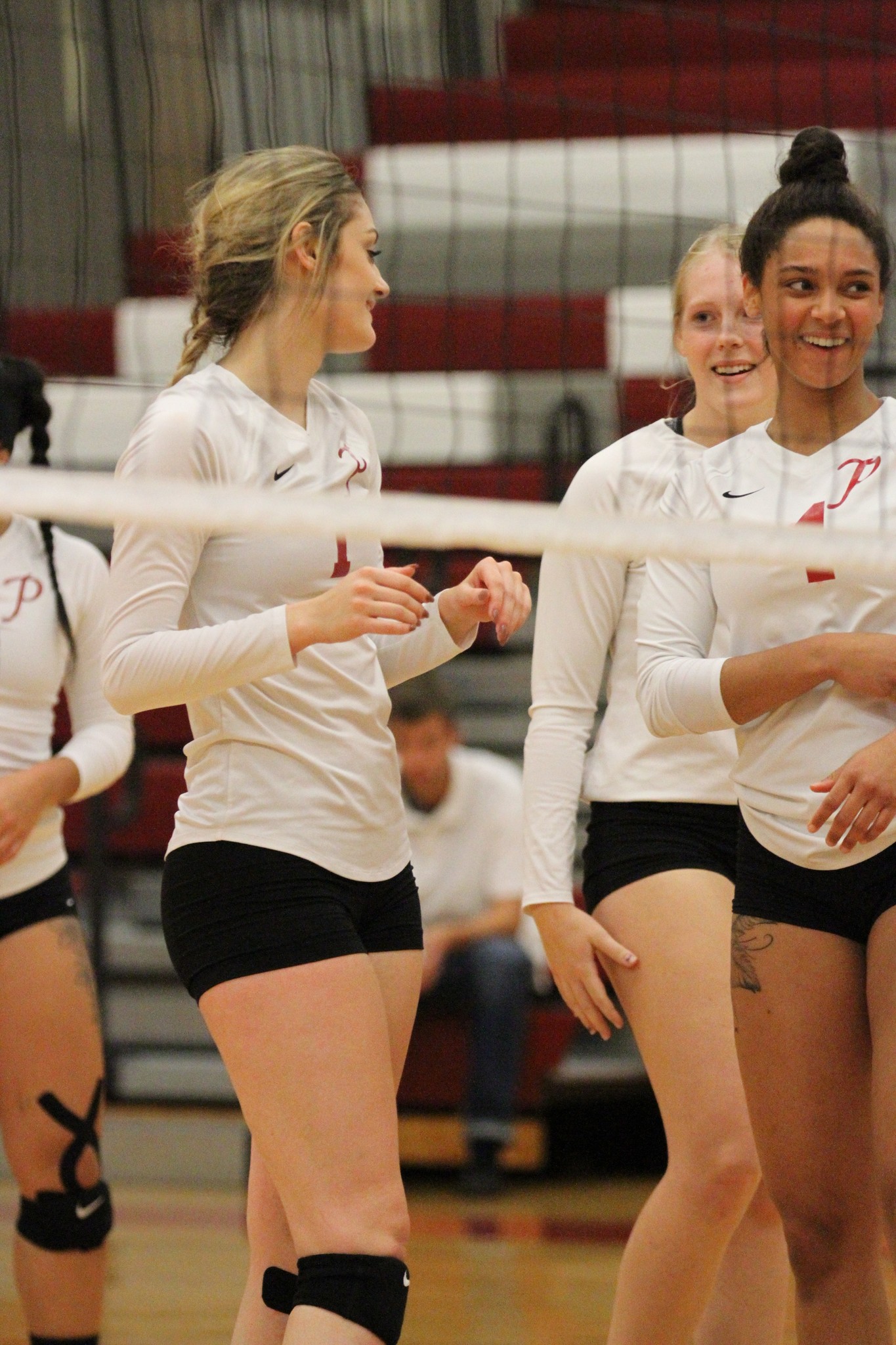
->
[387,680,552,1201]
[515,224,803,1345]
[633,126,895,1345]
[0,349,137,1345]
[101,145,533,1345]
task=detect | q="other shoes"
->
[460,1161,502,1195]
